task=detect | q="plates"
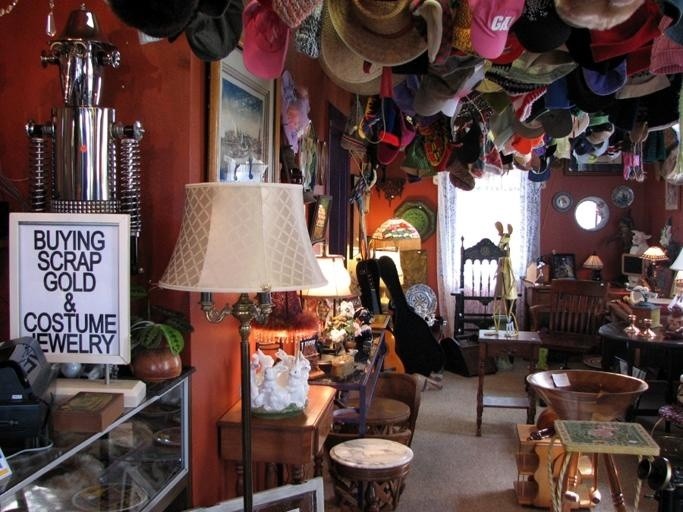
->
[72,479,147,511]
[404,284,436,321]
[152,428,182,447]
[137,396,178,415]
[391,201,435,244]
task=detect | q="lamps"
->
[638,247,670,298]
[582,250,603,281]
[369,246,404,305]
[296,254,359,316]
[157,183,328,512]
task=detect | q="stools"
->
[325,372,421,512]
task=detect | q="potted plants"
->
[132,316,185,381]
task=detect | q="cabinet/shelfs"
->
[513,424,595,508]
[0,368,198,512]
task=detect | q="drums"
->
[327,438,413,512]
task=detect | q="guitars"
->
[366,273,405,374]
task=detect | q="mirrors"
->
[574,195,611,232]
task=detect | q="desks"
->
[477,329,543,437]
[520,278,633,371]
[277,332,387,488]
[371,315,394,333]
[597,322,683,434]
[217,384,337,512]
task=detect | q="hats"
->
[242,0,289,79]
[185,0,244,61]
[292,0,325,59]
[108,0,198,43]
[271,0,321,28]
[320,0,683,191]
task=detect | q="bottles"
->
[641,318,653,337]
[624,314,639,335]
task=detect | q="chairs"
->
[451,235,517,341]
[529,278,610,406]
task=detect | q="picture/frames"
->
[564,159,624,175]
[209,37,280,183]
[8,212,131,365]
[309,195,333,244]
